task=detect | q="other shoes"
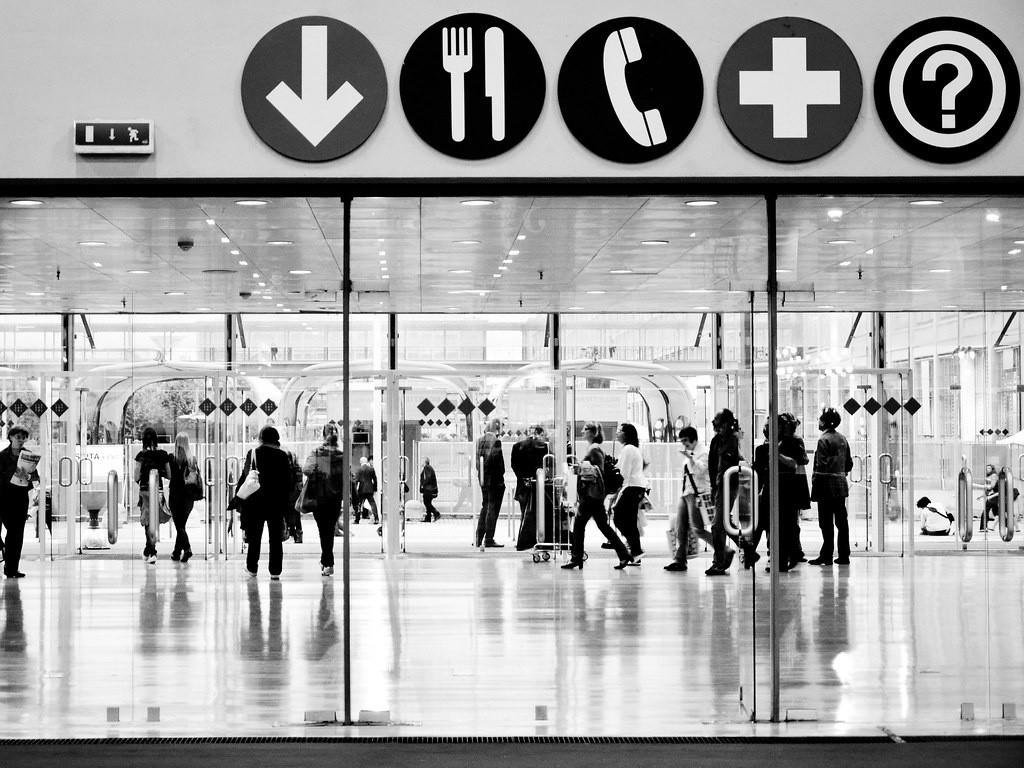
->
[245,564,257,577]
[375,517,378,524]
[631,550,850,574]
[420,520,430,522]
[181,550,192,562]
[433,513,441,522]
[147,556,156,563]
[5,569,25,578]
[363,515,370,519]
[321,566,333,575]
[170,555,180,560]
[485,541,504,547]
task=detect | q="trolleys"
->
[529,467,588,563]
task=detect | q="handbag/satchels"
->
[696,491,717,524]
[184,459,203,493]
[237,449,261,501]
[424,485,438,497]
[667,526,697,556]
[295,449,319,514]
[604,454,623,493]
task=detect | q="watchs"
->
[689,450,694,457]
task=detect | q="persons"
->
[168,432,199,563]
[561,422,635,570]
[134,427,172,565]
[0,424,41,578]
[33,486,58,542]
[976,464,1021,533]
[611,422,655,567]
[225,425,304,579]
[138,421,147,440]
[420,456,443,524]
[471,419,507,547]
[153,417,168,443]
[706,409,761,576]
[509,426,575,549]
[663,427,736,571]
[352,455,380,524]
[807,407,855,565]
[916,497,951,536]
[742,413,810,573]
[301,423,358,577]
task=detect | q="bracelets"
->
[27,474,31,480]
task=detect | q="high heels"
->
[561,560,583,569]
[614,554,634,569]
